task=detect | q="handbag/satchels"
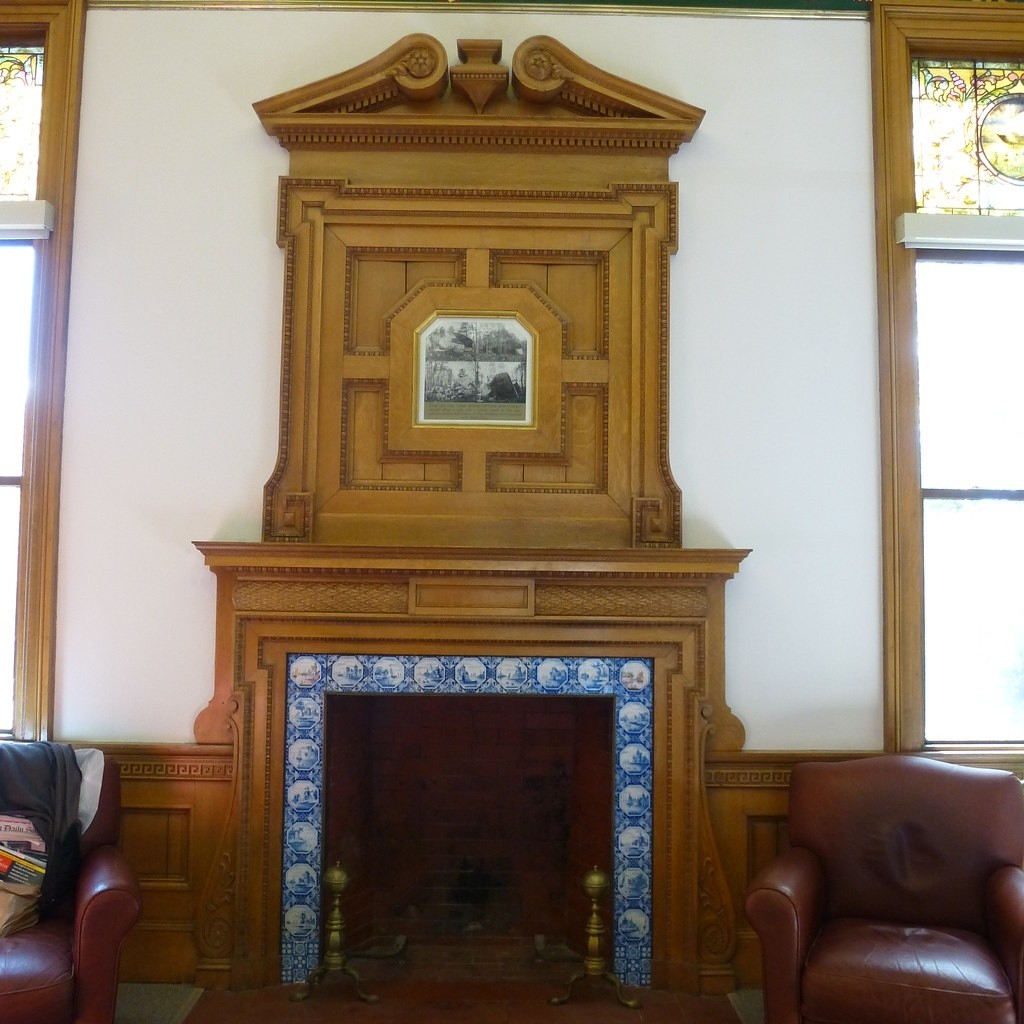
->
[0,881,42,938]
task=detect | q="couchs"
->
[0,742,143,1024]
[741,754,1023,1024]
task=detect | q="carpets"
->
[115,981,207,1024]
